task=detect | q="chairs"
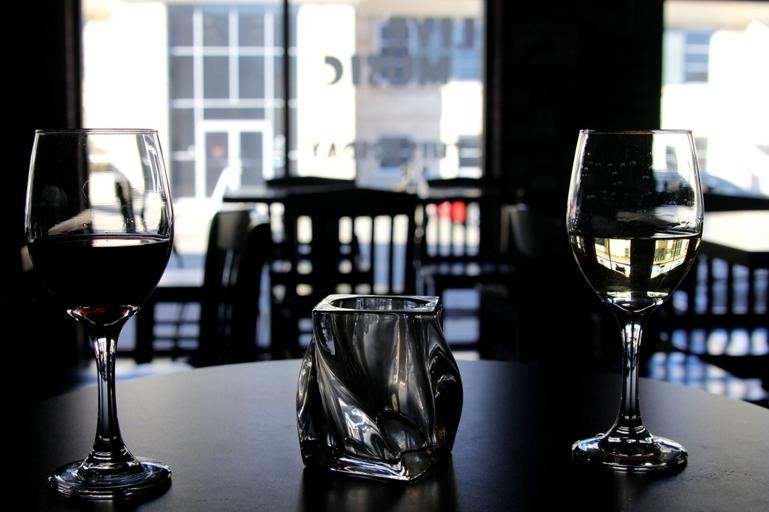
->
[408,173,767,404]
[264,175,358,306]
[138,210,253,366]
[264,191,418,363]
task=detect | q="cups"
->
[295,292,463,480]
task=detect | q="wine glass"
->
[566,127,705,473]
[24,127,174,499]
